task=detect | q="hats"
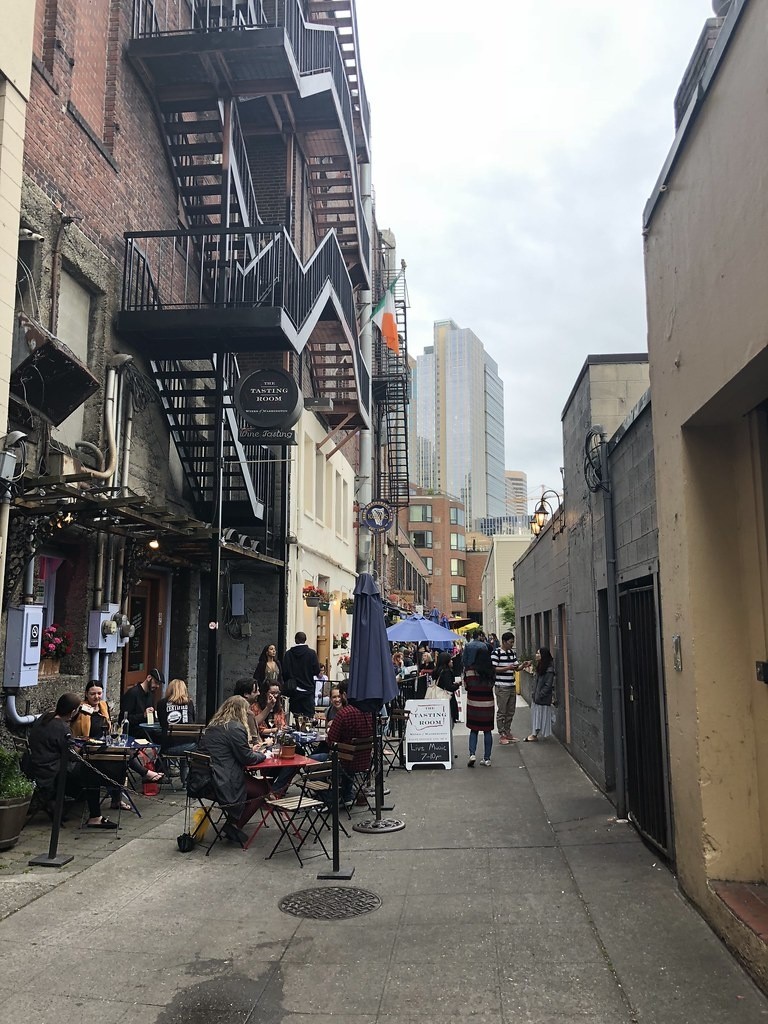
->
[150,668,165,684]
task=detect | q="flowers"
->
[333,635,339,644]
[341,633,350,643]
[304,585,334,602]
[336,655,351,664]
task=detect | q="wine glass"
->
[99,725,128,747]
[310,718,318,733]
[268,719,275,735]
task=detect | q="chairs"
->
[13,707,412,869]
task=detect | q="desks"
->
[78,744,159,819]
[140,723,205,781]
[272,728,326,753]
[242,755,321,850]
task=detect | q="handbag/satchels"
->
[284,679,314,695]
[177,833,194,852]
[191,807,211,840]
[424,669,453,700]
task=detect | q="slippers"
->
[142,772,164,783]
[110,804,131,810]
[523,737,538,742]
[87,817,117,829]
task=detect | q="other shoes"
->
[222,823,248,841]
[312,804,332,812]
[339,798,353,807]
[467,754,476,766]
[506,735,518,741]
[499,737,509,745]
[480,758,491,766]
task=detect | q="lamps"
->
[535,491,563,533]
[530,500,556,540]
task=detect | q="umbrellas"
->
[347,574,407,832]
[428,606,445,651]
[439,616,454,650]
[386,613,463,692]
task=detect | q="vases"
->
[342,643,345,646]
[320,603,329,611]
[334,645,338,649]
[341,664,350,672]
[306,597,319,608]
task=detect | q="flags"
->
[369,278,400,356]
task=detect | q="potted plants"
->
[0,691,36,852]
[343,599,354,614]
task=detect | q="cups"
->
[272,748,281,757]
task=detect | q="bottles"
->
[121,711,129,734]
[394,665,405,679]
[250,735,271,759]
[296,712,318,733]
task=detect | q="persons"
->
[490,632,532,745]
[26,694,118,828]
[523,648,555,743]
[158,680,195,791]
[305,679,373,812]
[314,663,329,695]
[252,643,284,684]
[280,632,320,716]
[465,649,496,766]
[392,630,500,690]
[311,687,343,793]
[187,695,271,842]
[248,682,303,803]
[231,677,274,750]
[120,669,172,784]
[72,680,164,811]
